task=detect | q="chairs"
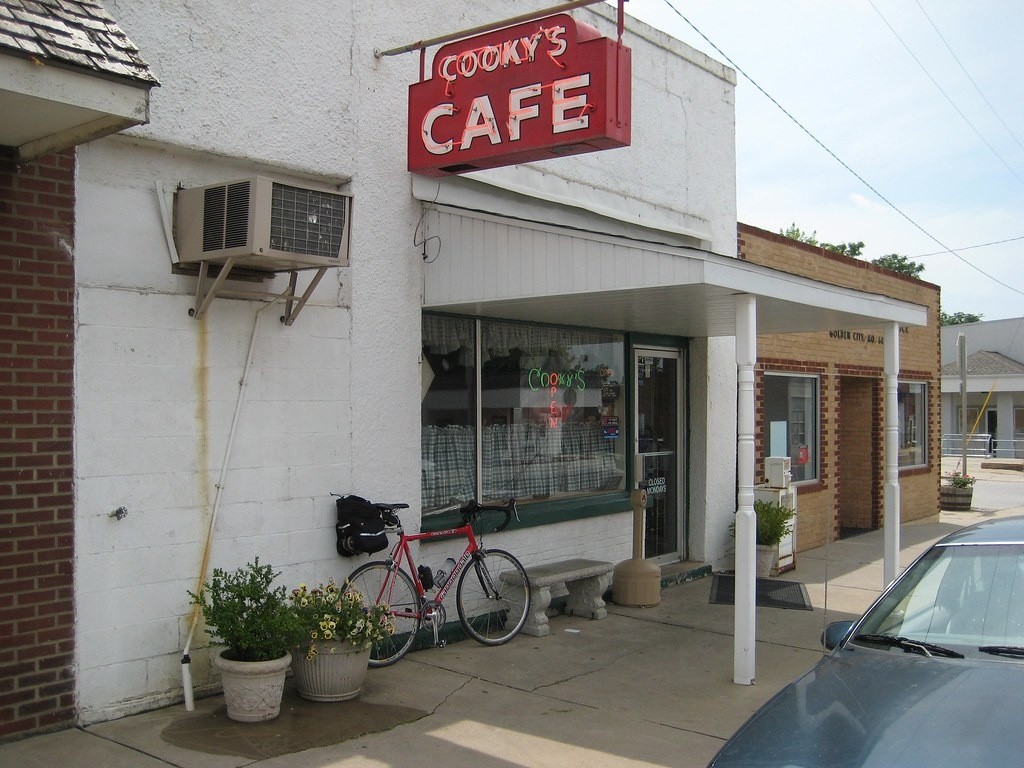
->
[989,564,1024,637]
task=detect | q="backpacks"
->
[336,495,388,557]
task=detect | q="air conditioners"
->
[177,175,356,273]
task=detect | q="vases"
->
[940,485,974,512]
[290,638,372,704]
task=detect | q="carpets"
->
[706,574,814,612]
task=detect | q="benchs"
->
[498,558,615,638]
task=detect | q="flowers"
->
[943,470,976,488]
[287,577,398,662]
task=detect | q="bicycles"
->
[336,497,531,669]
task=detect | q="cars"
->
[707,514,1024,767]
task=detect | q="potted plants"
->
[728,498,798,579]
[185,554,314,724]
